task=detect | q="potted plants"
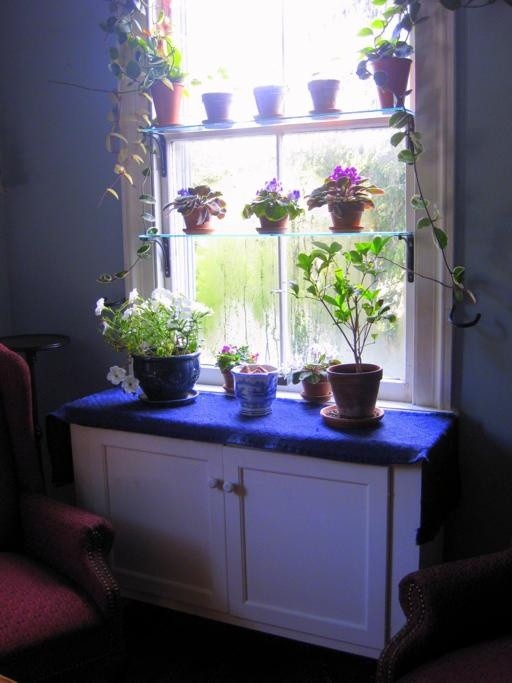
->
[272,236,402,428]
[357,0,479,306]
[216,342,263,367]
[291,353,344,403]
[95,0,187,283]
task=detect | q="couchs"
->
[1,344,121,671]
[375,547,511,682]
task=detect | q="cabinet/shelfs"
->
[69,383,458,660]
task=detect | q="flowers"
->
[161,185,227,217]
[242,176,306,201]
[94,286,213,353]
[305,166,383,199]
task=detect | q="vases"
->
[329,200,367,232]
[129,351,199,399]
[198,92,236,128]
[223,363,281,415]
[306,80,339,117]
[183,208,209,228]
[221,370,235,394]
[252,86,283,125]
[257,206,290,233]
